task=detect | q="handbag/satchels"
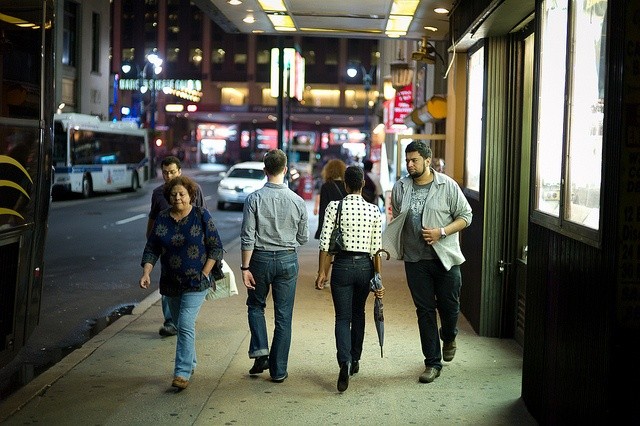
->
[328,200,347,256]
[211,260,227,281]
[206,259,238,301]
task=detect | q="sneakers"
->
[159,324,178,336]
[249,356,270,374]
[350,361,360,376]
[171,376,190,389]
[337,361,350,392]
[271,373,288,383]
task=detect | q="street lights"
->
[346,61,373,162]
[145,51,164,176]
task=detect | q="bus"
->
[51,112,151,200]
[0,0,59,368]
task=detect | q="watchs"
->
[440,227,446,239]
[240,264,250,270]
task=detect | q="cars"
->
[216,160,268,211]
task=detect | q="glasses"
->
[161,170,177,175]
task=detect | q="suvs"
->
[285,144,317,181]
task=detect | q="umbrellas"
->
[369,249,390,358]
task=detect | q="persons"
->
[240,147,310,382]
[315,166,382,392]
[140,176,223,388]
[382,140,473,382]
[362,162,386,207]
[145,156,209,335]
[314,159,349,283]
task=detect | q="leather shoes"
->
[419,367,440,383]
[443,337,457,362]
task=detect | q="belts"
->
[335,255,367,259]
